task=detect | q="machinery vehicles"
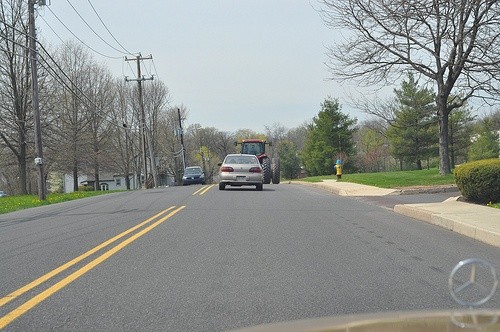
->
[233,139,280,184]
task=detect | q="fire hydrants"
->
[335,160,342,182]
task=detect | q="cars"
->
[0,191,8,198]
[217,153,264,191]
[181,166,206,186]
